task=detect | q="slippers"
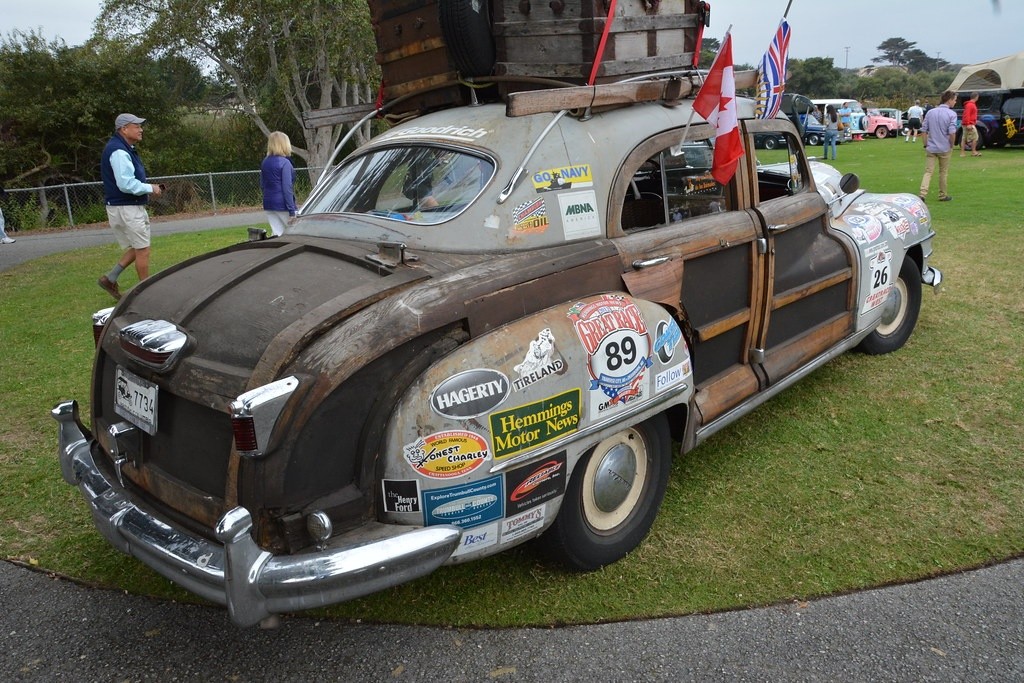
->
[971,152,981,157]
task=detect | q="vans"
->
[805,98,866,136]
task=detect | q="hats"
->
[115,113,147,130]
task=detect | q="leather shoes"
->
[98,274,125,301]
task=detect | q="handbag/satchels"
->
[837,114,844,131]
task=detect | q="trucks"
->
[942,54,1024,151]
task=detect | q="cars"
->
[749,114,826,149]
[49,83,942,632]
[900,111,911,131]
[865,107,903,139]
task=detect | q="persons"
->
[960,91,981,157]
[822,105,844,160]
[0,185,16,244]
[838,102,851,127]
[260,131,299,237]
[905,99,923,142]
[403,148,449,208]
[98,113,167,301]
[918,90,958,202]
[811,105,823,123]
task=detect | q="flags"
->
[693,33,744,186]
[755,17,792,120]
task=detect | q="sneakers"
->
[1,236,16,245]
[938,195,953,202]
[920,195,924,203]
